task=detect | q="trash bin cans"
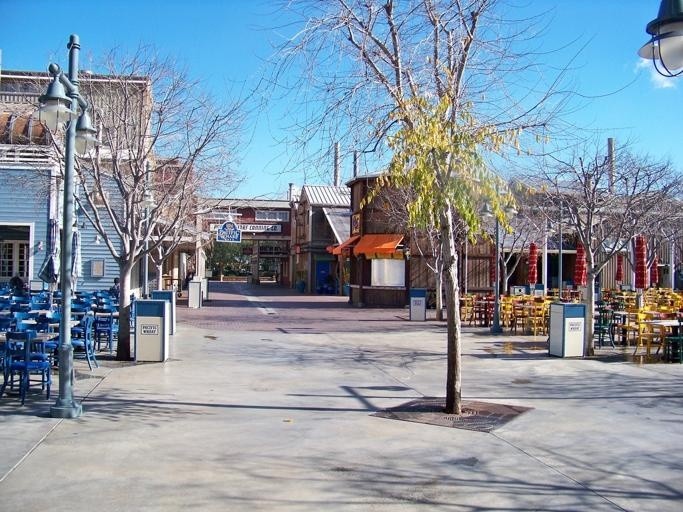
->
[296,280,305,294]
[134,289,177,362]
[343,283,350,296]
[550,302,587,358]
[188,282,201,309]
[409,288,427,321]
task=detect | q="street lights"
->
[36,33,98,419]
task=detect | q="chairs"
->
[0,283,135,406]
[458,282,682,363]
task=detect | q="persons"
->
[11,273,24,289]
[111,278,121,298]
